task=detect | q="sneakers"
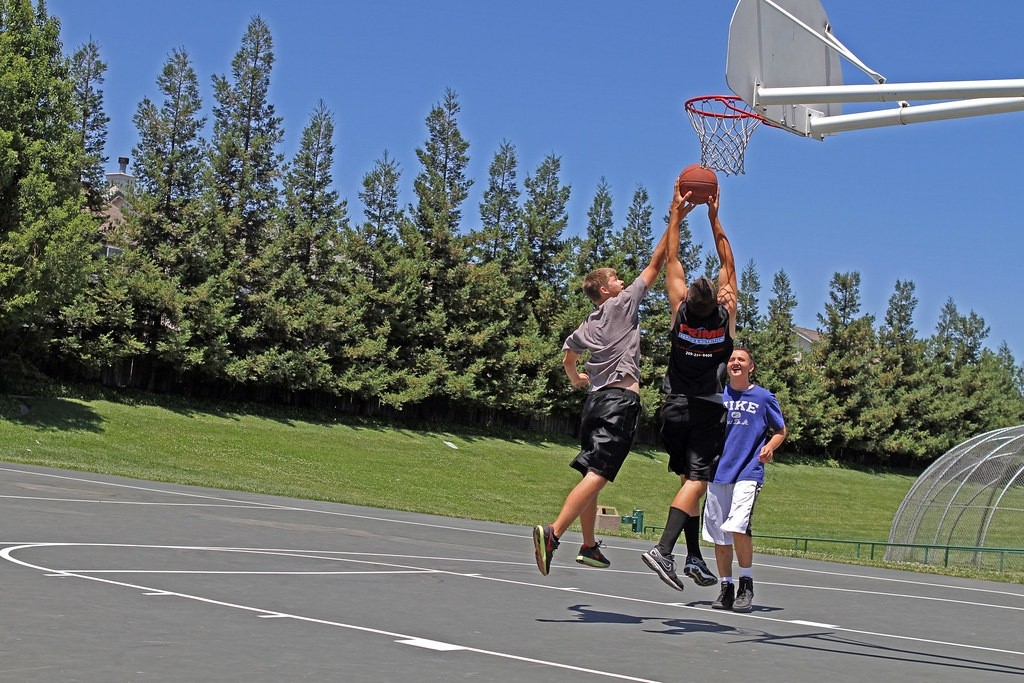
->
[641,546,684,591]
[712,581,735,609]
[684,555,718,586]
[732,576,754,613]
[533,525,560,576]
[575,540,610,568]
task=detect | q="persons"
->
[533,204,697,577]
[702,347,786,613]
[643,177,738,592]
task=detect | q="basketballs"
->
[678,163,718,204]
